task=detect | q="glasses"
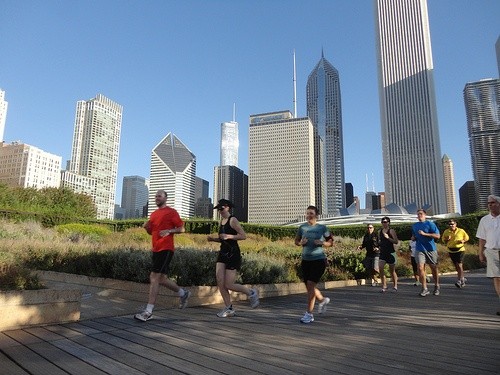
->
[488,201,496,204]
[381,221,388,223]
[218,208,224,211]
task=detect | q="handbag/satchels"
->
[448,246,463,251]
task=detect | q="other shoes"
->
[426,276,431,283]
[415,280,420,287]
[299,311,314,324]
[217,304,236,318]
[248,289,260,309]
[178,289,190,310]
[317,297,330,315]
[455,279,465,289]
[379,287,388,293]
[391,288,399,293]
[135,311,153,322]
[433,289,441,296]
[496,311,500,316]
[420,288,431,297]
[372,282,379,287]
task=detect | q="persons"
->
[411,209,442,296]
[410,235,432,285]
[131,190,191,322]
[295,205,334,324]
[442,219,469,288]
[378,216,398,293]
[205,199,259,318]
[360,223,382,287]
[476,195,500,316]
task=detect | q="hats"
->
[213,198,233,209]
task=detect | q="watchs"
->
[168,229,172,234]
